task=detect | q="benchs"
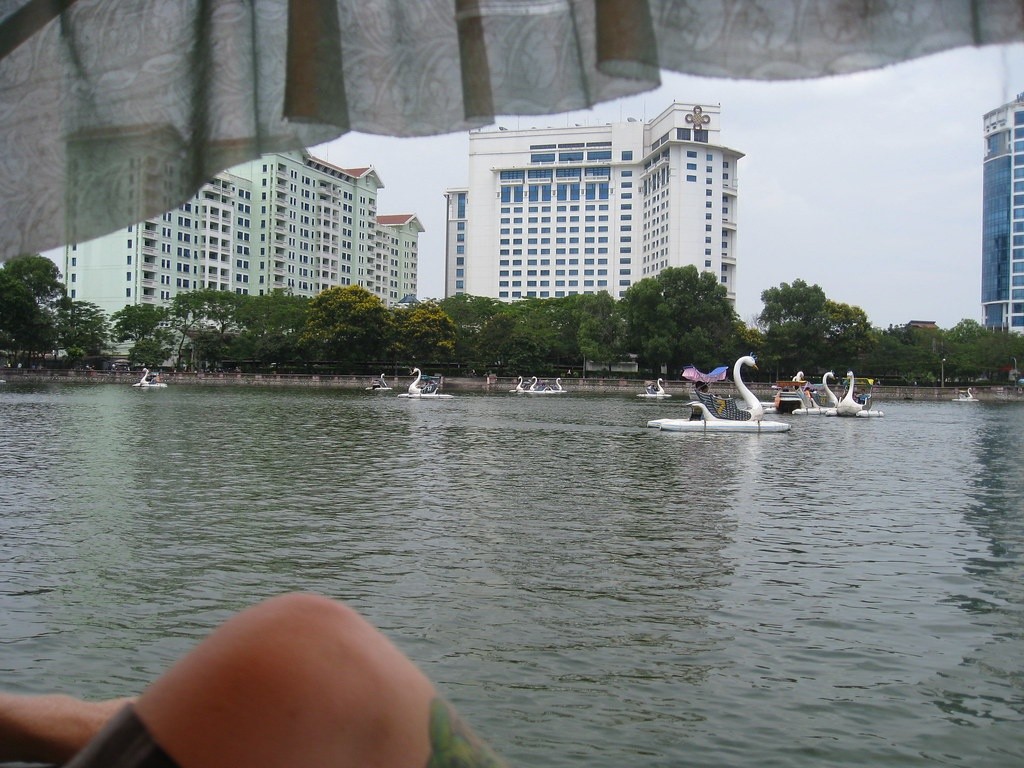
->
[780,391,804,399]
[689,390,739,419]
[860,393,871,406]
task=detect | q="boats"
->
[647,354,790,433]
[364,373,394,392]
[132,367,169,388]
[759,370,886,417]
[398,367,453,399]
[635,377,672,399]
[508,376,568,395]
[950,387,980,401]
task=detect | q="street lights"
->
[942,358,946,388]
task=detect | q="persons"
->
[804,387,817,395]
[0,590,505,768]
[696,381,710,393]
[859,389,868,401]
